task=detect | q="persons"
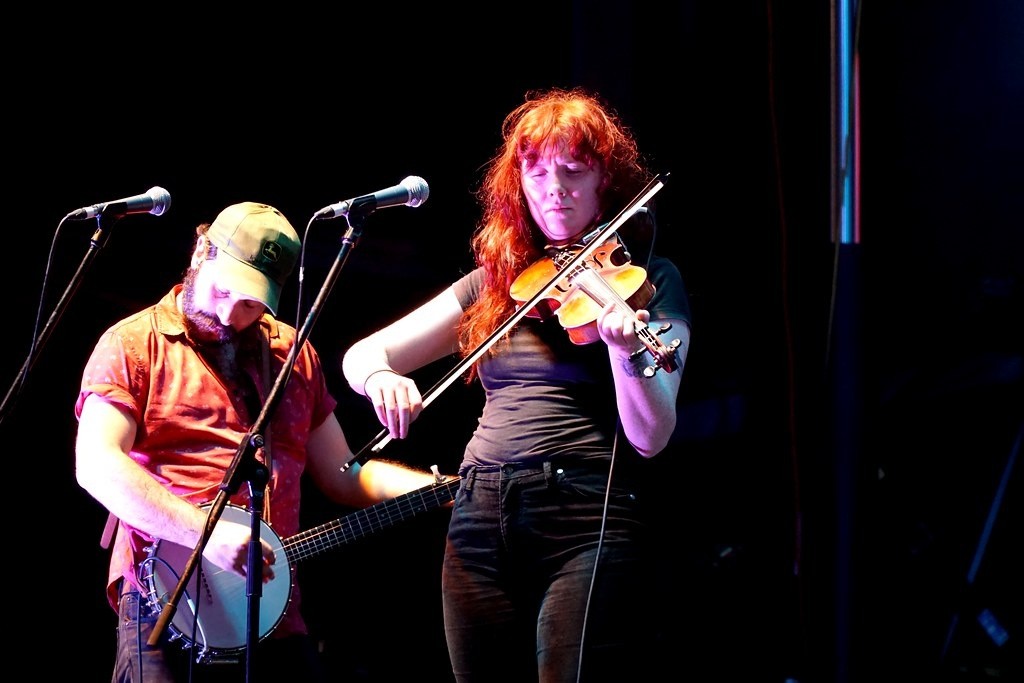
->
[74,201,459,683]
[342,94,692,682]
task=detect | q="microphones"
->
[314,175,430,221]
[69,187,171,221]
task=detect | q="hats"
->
[206,201,301,317]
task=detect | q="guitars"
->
[147,477,463,658]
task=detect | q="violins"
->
[508,233,684,380]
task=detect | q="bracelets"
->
[364,370,399,402]
